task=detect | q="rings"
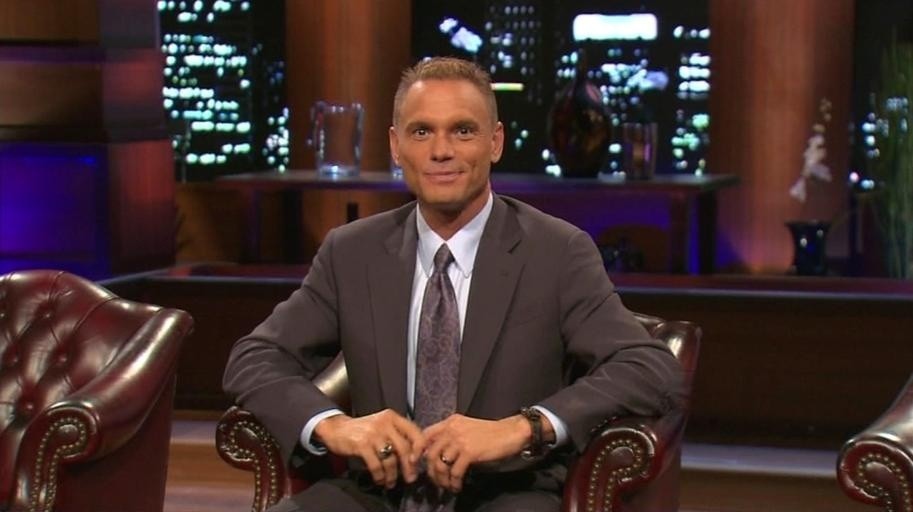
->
[378,442,391,459]
[439,449,453,467]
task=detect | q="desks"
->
[214,168,742,275]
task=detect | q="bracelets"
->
[519,405,544,458]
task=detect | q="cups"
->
[620,120,661,181]
[307,102,364,179]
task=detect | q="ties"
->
[399,244,459,512]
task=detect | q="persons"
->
[219,54,683,511]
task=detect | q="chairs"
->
[0,268,196,511]
[214,308,705,511]
